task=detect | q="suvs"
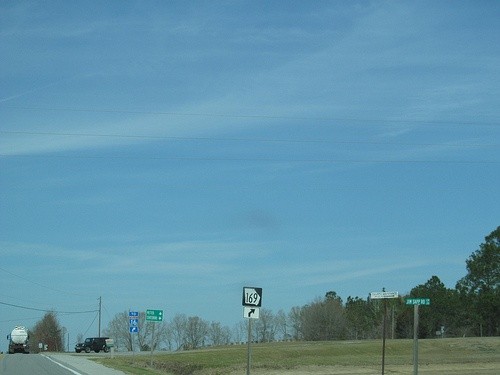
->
[73,336,111,354]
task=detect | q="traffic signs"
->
[144,308,163,322]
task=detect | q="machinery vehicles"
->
[6,325,32,355]
